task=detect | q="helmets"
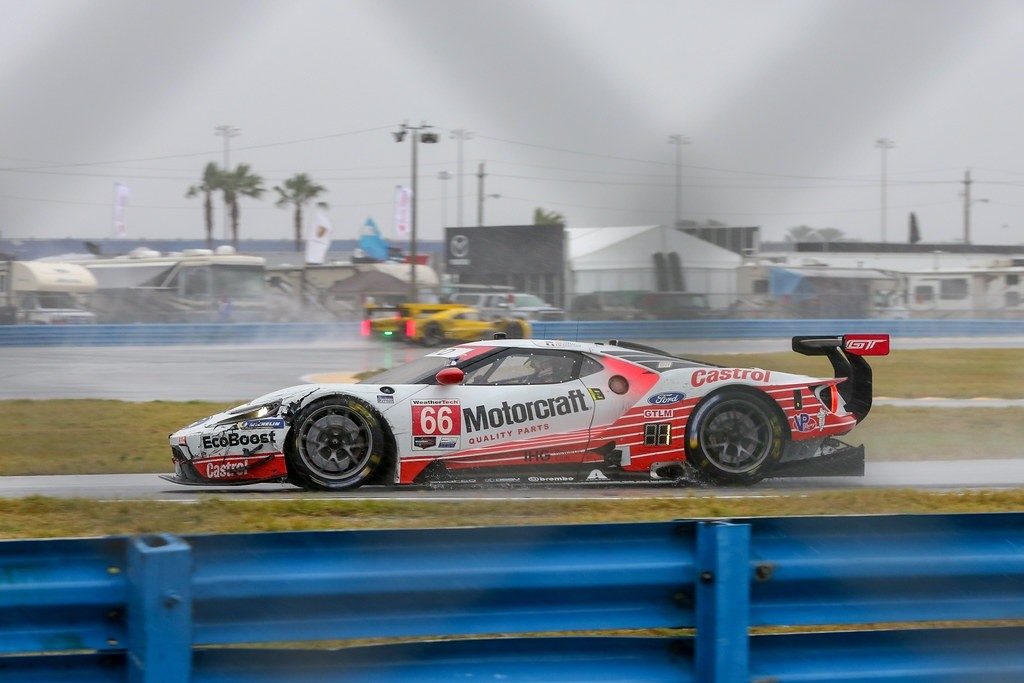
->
[523,355,553,378]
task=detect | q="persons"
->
[527,355,553,383]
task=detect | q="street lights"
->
[390,122,437,296]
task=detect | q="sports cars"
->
[157,327,890,494]
[1,245,566,352]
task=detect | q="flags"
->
[303,215,334,265]
[357,218,388,261]
